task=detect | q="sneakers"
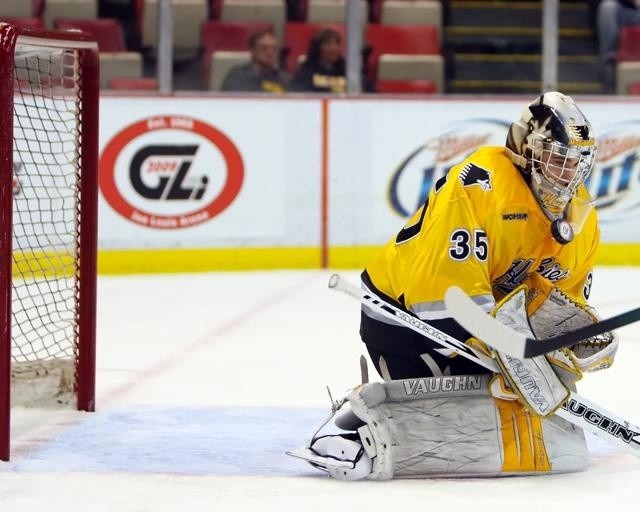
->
[308,432,372,479]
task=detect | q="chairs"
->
[3,1,448,91]
[615,24,640,97]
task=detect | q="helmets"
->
[505,92,601,234]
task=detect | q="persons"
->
[306,93,620,482]
[585,0,640,95]
[215,29,304,96]
[286,28,377,92]
